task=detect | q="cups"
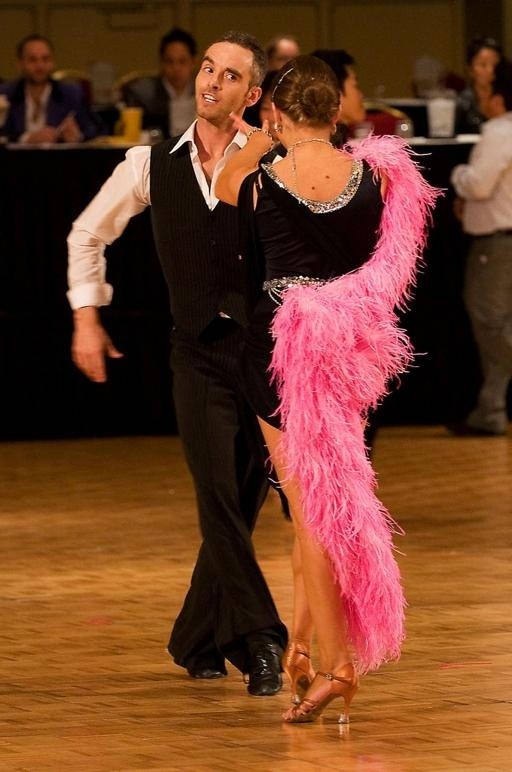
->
[425,91,458,138]
[120,109,144,142]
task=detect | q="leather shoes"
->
[247,641,283,696]
[188,658,227,678]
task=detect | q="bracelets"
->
[245,129,274,156]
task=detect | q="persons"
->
[1,25,365,146]
[64,28,291,697]
[212,52,435,725]
[445,56,512,438]
[453,40,504,133]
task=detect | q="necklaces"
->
[286,136,336,157]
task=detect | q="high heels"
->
[282,640,358,724]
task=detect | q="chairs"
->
[107,65,161,135]
[50,68,94,109]
[347,101,417,141]
